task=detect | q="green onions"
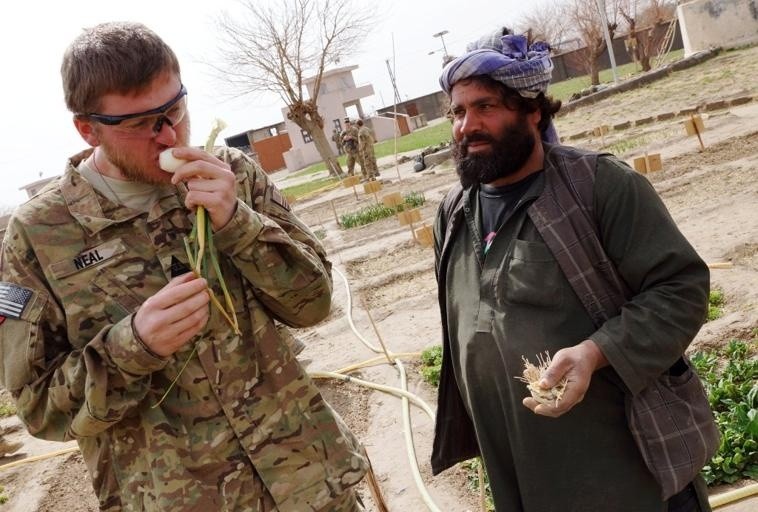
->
[143,117,241,410]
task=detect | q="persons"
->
[354,119,381,184]
[340,117,366,182]
[0,20,370,511]
[331,128,345,157]
[427,25,724,511]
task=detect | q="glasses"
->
[76,84,189,136]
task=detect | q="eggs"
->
[158,147,186,173]
